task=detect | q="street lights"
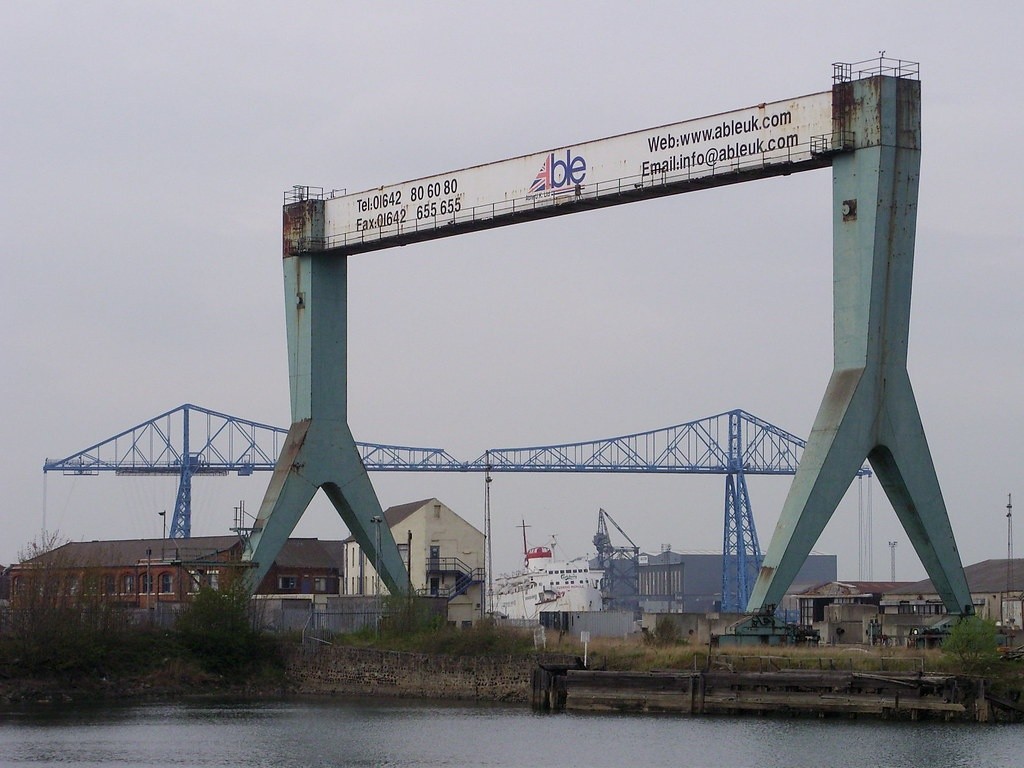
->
[370,515,384,596]
[889,541,898,583]
[158,509,166,559]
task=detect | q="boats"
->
[484,518,602,620]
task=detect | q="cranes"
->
[40,404,874,623]
